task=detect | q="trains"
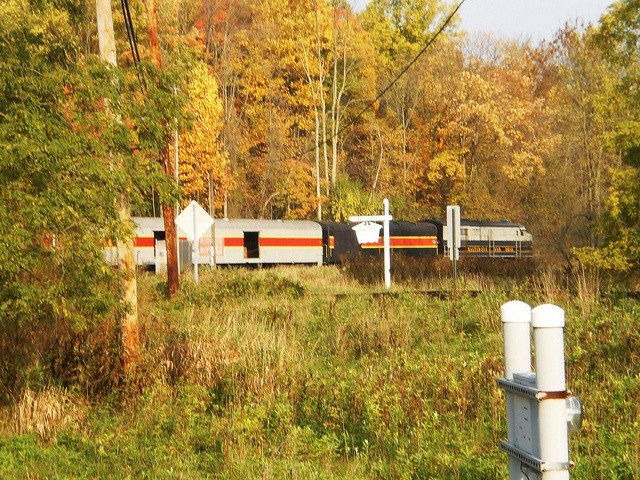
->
[37,216,535,270]
